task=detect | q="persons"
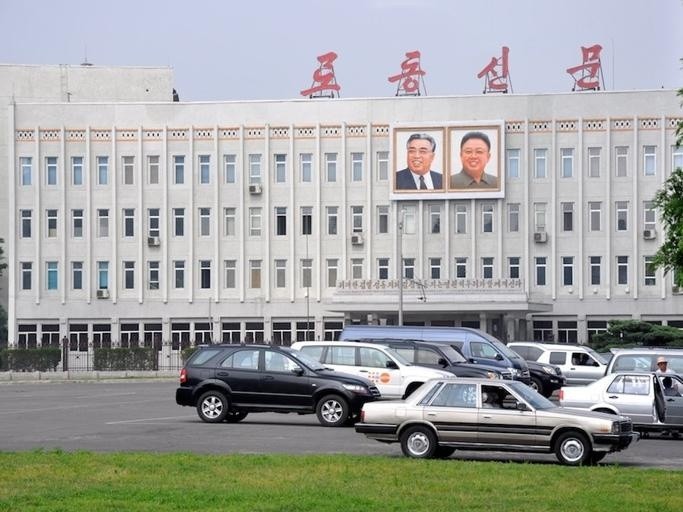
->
[396,133,442,190]
[450,131,497,188]
[576,353,682,436]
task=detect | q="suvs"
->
[175,343,382,427]
[290,337,514,406]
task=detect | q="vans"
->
[340,324,529,401]
[605,348,682,391]
[507,342,608,386]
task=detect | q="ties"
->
[419,176,427,189]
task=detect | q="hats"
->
[657,357,667,365]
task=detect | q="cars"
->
[526,360,565,398]
[355,378,631,466]
[559,370,683,435]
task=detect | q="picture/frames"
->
[391,125,502,194]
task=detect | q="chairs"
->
[573,357,579,364]
[481,390,500,409]
